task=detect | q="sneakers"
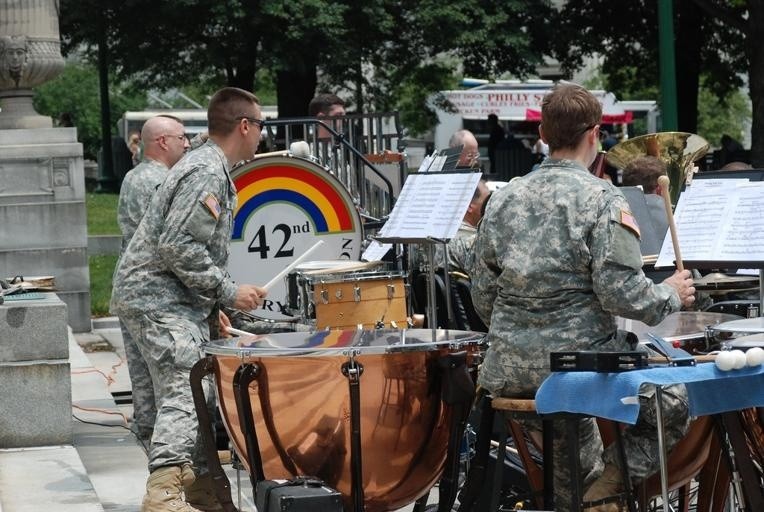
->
[217,450,232,464]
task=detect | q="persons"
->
[106,85,268,512]
[448,130,479,169]
[485,112,505,173]
[468,84,697,512]
[431,179,492,270]
[112,114,191,288]
[621,154,669,197]
[600,131,624,187]
[306,94,362,210]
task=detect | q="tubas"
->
[605,131,709,212]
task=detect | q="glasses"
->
[236,116,264,130]
[463,152,480,159]
[156,134,187,143]
[583,125,609,142]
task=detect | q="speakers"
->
[457,435,544,512]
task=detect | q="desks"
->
[536,364,764,510]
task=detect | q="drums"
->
[617,309,763,501]
[286,259,409,332]
[200,327,489,509]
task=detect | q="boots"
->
[183,472,239,512]
[142,466,204,512]
[582,463,631,512]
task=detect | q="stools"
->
[490,393,637,512]
